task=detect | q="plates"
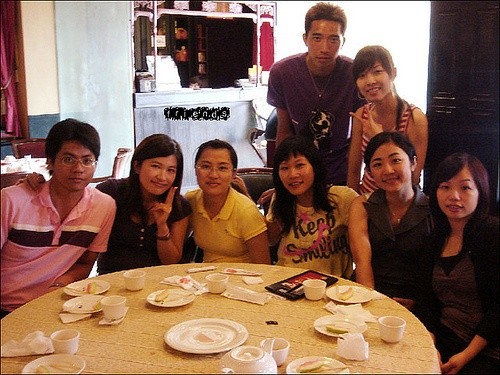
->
[285,356,350,374]
[62,294,106,313]
[326,285,373,303]
[164,318,248,354]
[63,279,110,296]
[22,354,86,375]
[148,289,195,308]
[314,314,367,337]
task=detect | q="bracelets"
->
[156,230,172,241]
[49,282,67,287]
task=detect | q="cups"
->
[50,328,79,355]
[206,273,229,293]
[378,315,406,343]
[303,280,326,301]
[101,294,126,321]
[260,338,290,366]
[123,270,144,291]
[2,155,40,174]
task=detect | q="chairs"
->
[90,144,133,185]
[236,164,274,205]
[11,138,50,158]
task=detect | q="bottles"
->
[136,70,155,94]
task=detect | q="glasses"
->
[194,163,235,174]
[53,157,98,168]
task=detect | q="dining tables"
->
[0,261,440,374]
[0,154,53,183]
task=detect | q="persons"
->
[394,151,500,375]
[182,140,271,264]
[15,133,192,276]
[226,135,375,292]
[0,119,117,319]
[346,45,429,198]
[255,2,369,205]
[348,132,437,308]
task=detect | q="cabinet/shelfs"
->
[170,15,210,88]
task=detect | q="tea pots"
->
[217,335,277,374]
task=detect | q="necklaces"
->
[305,55,335,98]
